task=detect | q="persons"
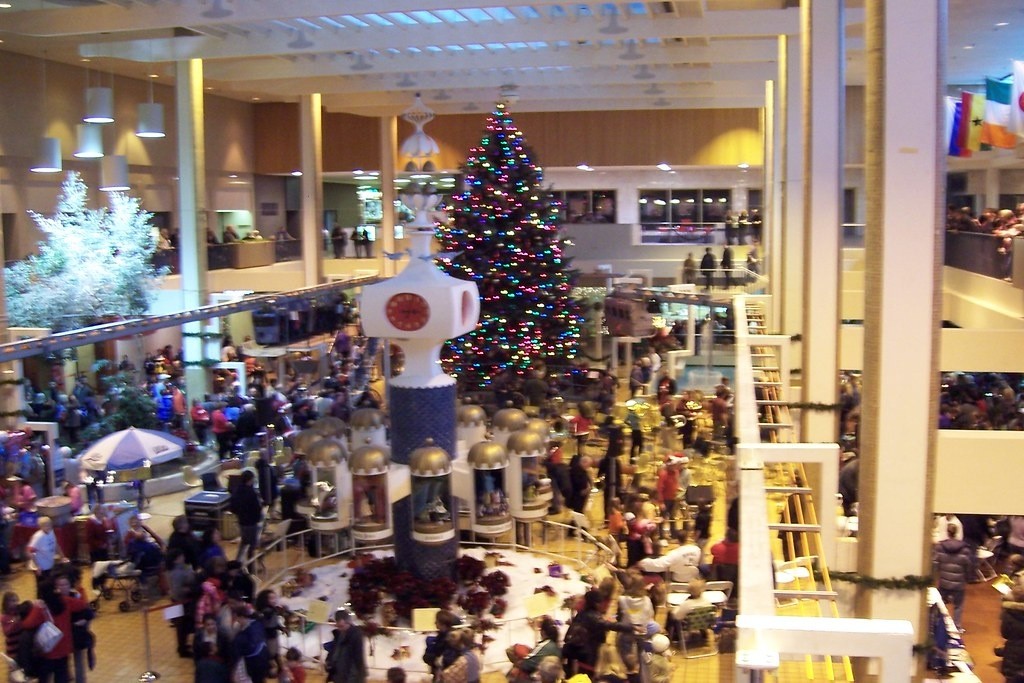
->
[0,204,1024,682]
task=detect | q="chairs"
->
[254,518,293,575]
[117,508,168,596]
[671,605,719,659]
[707,581,735,598]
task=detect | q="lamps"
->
[98,34,132,193]
[72,35,106,158]
[30,50,63,174]
[132,29,168,138]
[81,33,116,124]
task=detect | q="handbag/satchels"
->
[34,605,63,653]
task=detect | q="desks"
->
[228,240,275,269]
[10,522,76,564]
[153,244,231,274]
[271,238,327,264]
[667,591,726,609]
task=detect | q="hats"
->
[651,634,670,654]
[664,455,679,466]
[675,453,689,463]
[646,621,661,636]
[585,591,608,604]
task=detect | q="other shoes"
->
[958,628,966,634]
